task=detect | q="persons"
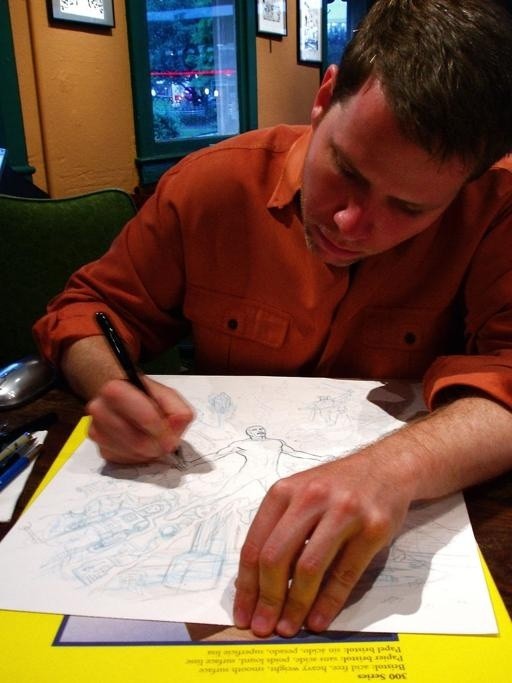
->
[30,0,511,638]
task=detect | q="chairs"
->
[0,188,171,370]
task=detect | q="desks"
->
[3,372,512,683]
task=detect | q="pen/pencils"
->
[0,412,58,490]
[92,310,187,464]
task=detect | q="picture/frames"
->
[256,0,323,69]
[49,0,114,24]
